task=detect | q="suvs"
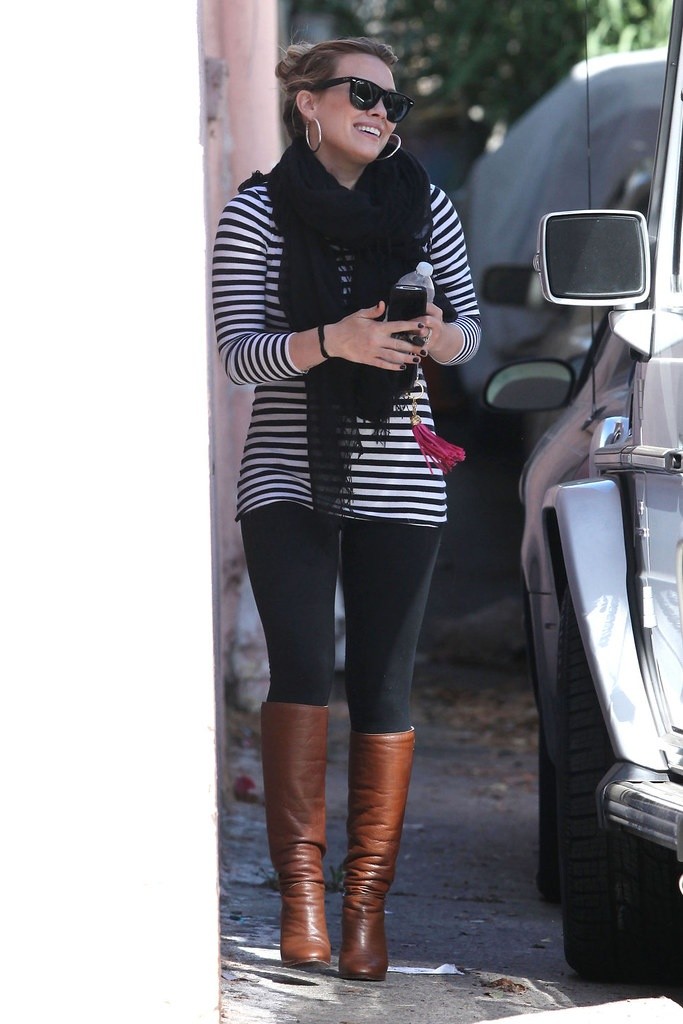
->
[475,1,683,1011]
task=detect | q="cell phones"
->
[386,285,427,335]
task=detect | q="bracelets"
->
[317,322,332,359]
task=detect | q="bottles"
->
[383,262,436,323]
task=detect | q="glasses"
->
[307,73,415,125]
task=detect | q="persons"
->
[210,40,481,973]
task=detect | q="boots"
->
[260,702,334,972]
[340,723,416,988]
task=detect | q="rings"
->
[422,328,432,343]
